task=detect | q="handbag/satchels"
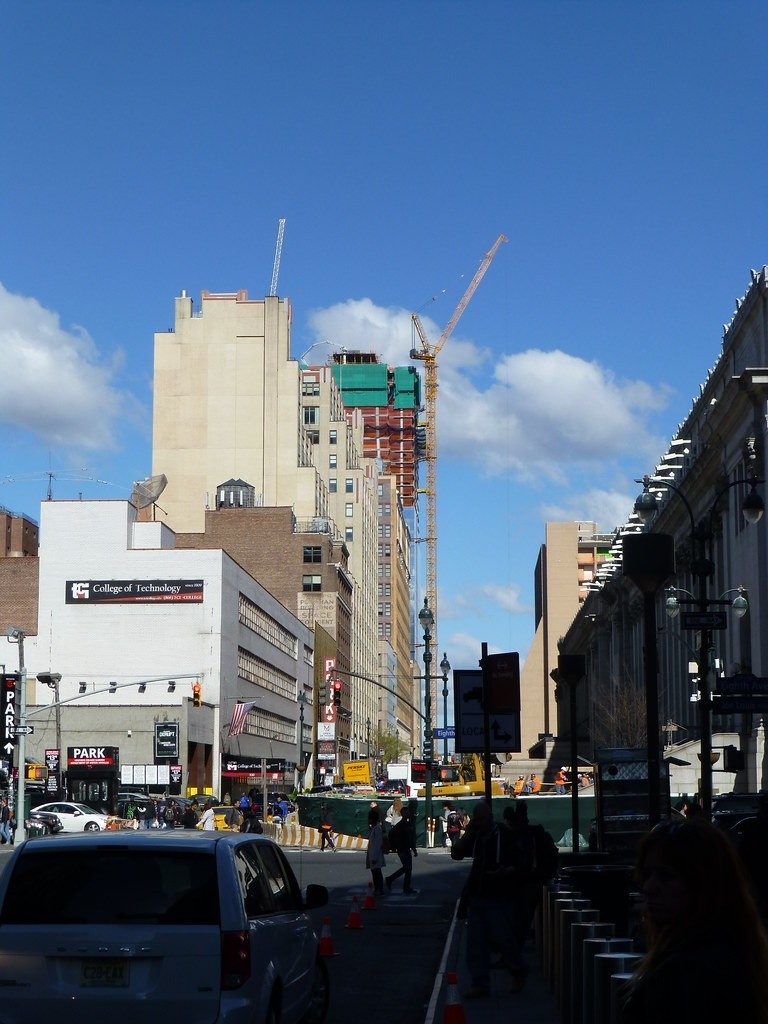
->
[382,830,390,854]
[450,826,460,833]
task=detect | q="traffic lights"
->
[24,763,49,781]
[319,681,331,706]
[423,737,433,763]
[127,729,132,738]
[333,682,342,706]
[192,683,202,708]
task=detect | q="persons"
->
[675,802,703,819]
[439,800,469,847]
[125,786,264,835]
[450,798,560,1000]
[0,799,11,844]
[318,802,337,852]
[512,766,593,797]
[618,817,768,1024]
[268,796,288,821]
[365,797,419,895]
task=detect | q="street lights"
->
[632,474,768,824]
[418,594,451,848]
[366,717,372,758]
[297,690,307,794]
[663,583,750,823]
[386,728,400,764]
[30,801,121,831]
[6,627,25,847]
[36,671,63,789]
[406,759,473,798]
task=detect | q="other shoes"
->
[510,975,526,992]
[404,888,417,894]
[373,888,385,895]
[463,986,489,999]
[386,877,392,891]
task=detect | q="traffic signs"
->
[9,724,35,734]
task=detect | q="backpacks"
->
[388,821,402,850]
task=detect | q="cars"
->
[0,830,330,1024]
[310,758,407,794]
[251,791,295,816]
[117,793,218,810]
[24,811,64,837]
[197,806,244,832]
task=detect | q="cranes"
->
[408,230,510,762]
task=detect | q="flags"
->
[228,700,258,738]
